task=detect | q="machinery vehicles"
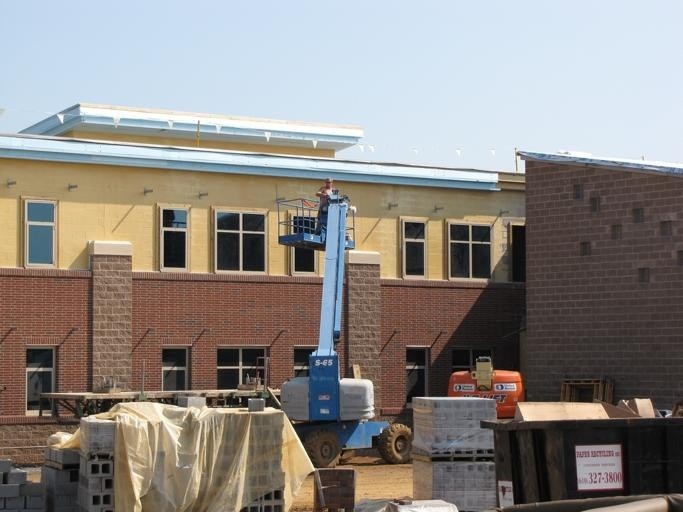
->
[444,352,532,421]
[272,185,414,472]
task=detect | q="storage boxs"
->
[314,468,354,512]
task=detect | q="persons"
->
[314,177,334,234]
